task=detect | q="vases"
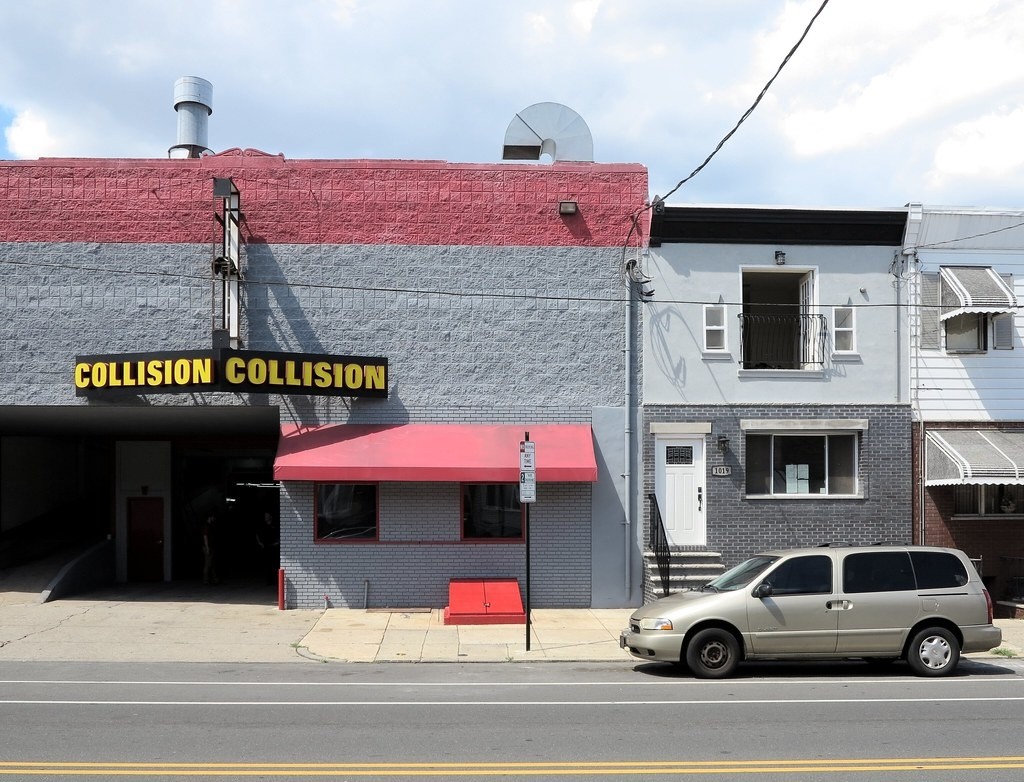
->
[1000,506,1017,513]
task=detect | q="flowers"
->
[1001,491,1016,508]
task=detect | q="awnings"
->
[941,267,1017,321]
[271,423,598,483]
[922,429,1024,486]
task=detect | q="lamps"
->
[775,250,786,265]
[558,201,578,215]
[718,435,730,455]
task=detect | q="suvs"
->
[618,542,1003,680]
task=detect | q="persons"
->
[256,512,280,586]
[199,514,223,586]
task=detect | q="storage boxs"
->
[786,464,809,494]
